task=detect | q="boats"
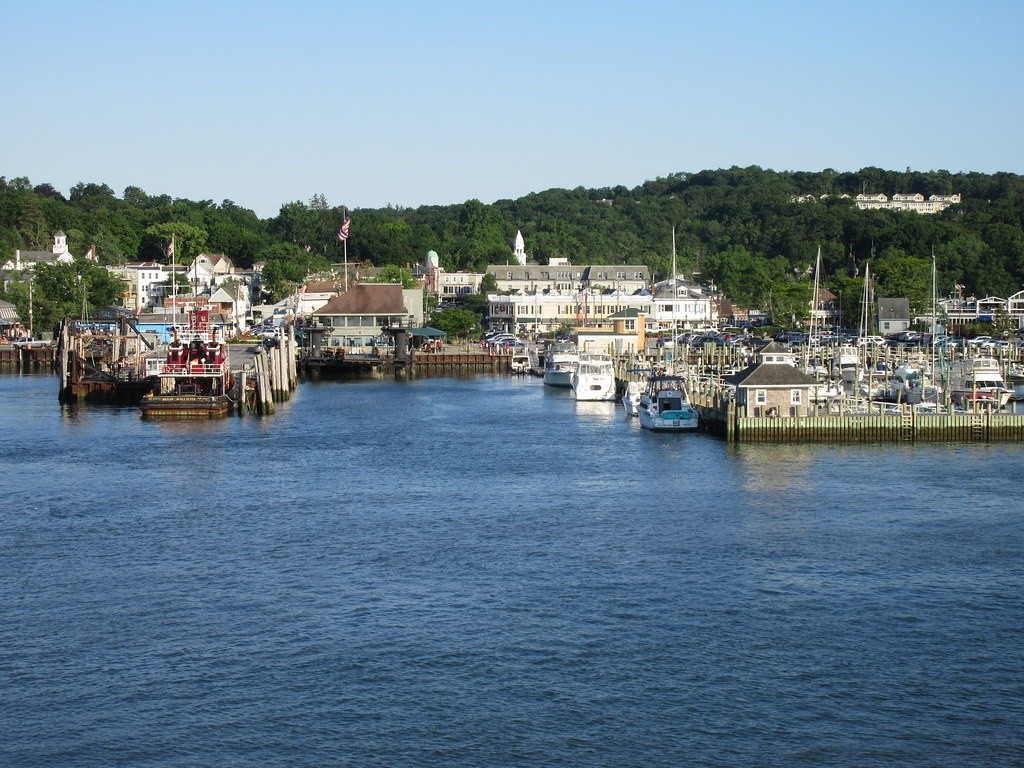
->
[1001,362,1024,386]
[636,371,699,432]
[951,369,1016,408]
[621,369,657,416]
[543,350,579,386]
[140,326,237,422]
[511,355,532,374]
[571,352,617,401]
[870,379,947,414]
[894,361,932,382]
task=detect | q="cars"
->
[663,318,886,357]
[887,327,1024,354]
[273,333,288,341]
[245,325,251,331]
[556,331,570,340]
[257,329,279,338]
[251,326,280,333]
[480,328,519,350]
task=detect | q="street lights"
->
[416,261,420,276]
[29,278,34,337]
